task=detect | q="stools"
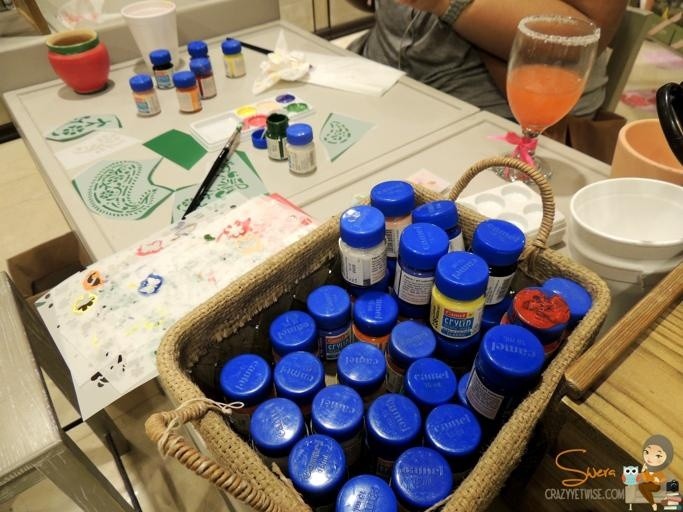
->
[0,270,142,512]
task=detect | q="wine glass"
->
[494,12,600,190]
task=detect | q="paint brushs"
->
[180,124,244,220]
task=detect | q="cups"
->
[609,116,683,187]
[563,175,683,344]
[119,1,180,73]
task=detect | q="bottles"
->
[126,37,244,125]
[260,113,316,179]
[205,175,593,512]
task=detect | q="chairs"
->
[314,0,653,117]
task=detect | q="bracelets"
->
[441,0,474,25]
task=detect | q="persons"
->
[345,0,631,125]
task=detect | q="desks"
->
[0,18,485,265]
[294,110,683,369]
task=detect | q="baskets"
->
[142,159,613,509]
